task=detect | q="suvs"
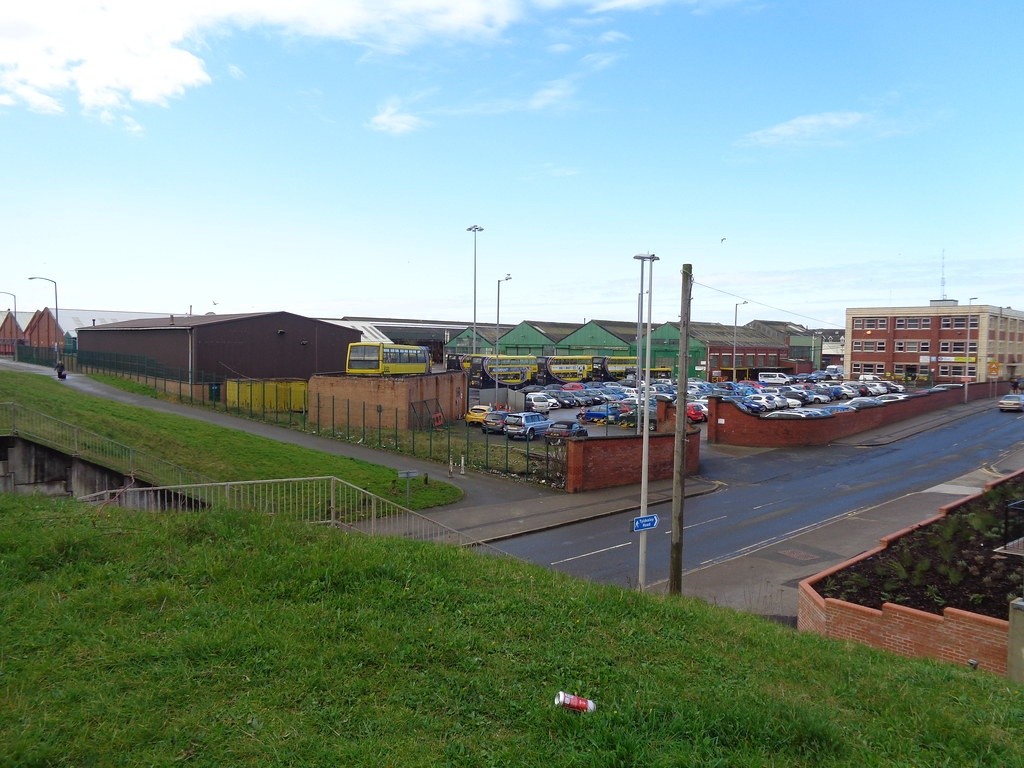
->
[503,412,555,440]
[544,421,588,445]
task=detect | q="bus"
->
[346,342,432,380]
[536,356,594,386]
[446,354,539,390]
[594,354,640,383]
[642,367,672,380]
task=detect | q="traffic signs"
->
[629,514,660,532]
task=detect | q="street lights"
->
[27,276,60,361]
[494,274,514,407]
[732,301,748,380]
[467,223,484,349]
[962,297,978,404]
[630,254,660,592]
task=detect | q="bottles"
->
[555,690,596,713]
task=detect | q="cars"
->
[481,411,513,436]
[465,405,494,427]
[524,364,963,431]
[997,393,1024,412]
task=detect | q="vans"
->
[758,372,793,387]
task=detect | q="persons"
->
[55,361,66,379]
[1010,378,1019,393]
